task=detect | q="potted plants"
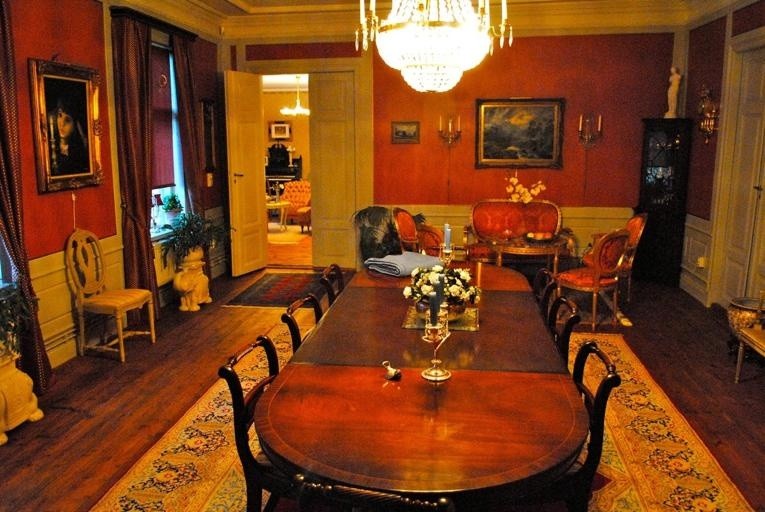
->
[157,215,230,314]
[164,195,184,220]
[0,284,46,446]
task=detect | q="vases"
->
[416,299,463,323]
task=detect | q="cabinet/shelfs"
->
[639,114,691,288]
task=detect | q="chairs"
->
[416,222,469,262]
[537,337,620,509]
[550,226,630,330]
[294,471,452,509]
[392,208,421,253]
[582,210,647,303]
[321,261,344,306]
[64,227,155,362]
[216,331,284,512]
[530,267,556,319]
[283,291,323,352]
[547,295,582,369]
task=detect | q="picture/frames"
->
[28,56,105,195]
[474,97,566,168]
[267,119,293,142]
[391,120,421,145]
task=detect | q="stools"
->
[734,328,765,384]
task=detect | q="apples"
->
[527,232,552,240]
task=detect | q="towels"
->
[363,251,444,278]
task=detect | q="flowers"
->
[400,263,482,305]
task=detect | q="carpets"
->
[90,322,757,510]
[221,271,355,310]
[267,222,311,244]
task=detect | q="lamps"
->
[281,75,310,118]
[353,0,515,94]
[694,83,718,145]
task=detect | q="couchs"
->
[277,180,312,225]
[462,199,562,267]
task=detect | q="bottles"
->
[760,289,765,331]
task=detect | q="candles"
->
[433,281,440,313]
[457,115,461,131]
[438,275,444,305]
[445,223,449,243]
[449,118,452,132]
[597,115,603,131]
[578,114,583,131]
[446,229,451,250]
[430,291,438,326]
[438,115,442,131]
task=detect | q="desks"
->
[266,202,291,231]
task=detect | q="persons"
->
[48,86,88,173]
